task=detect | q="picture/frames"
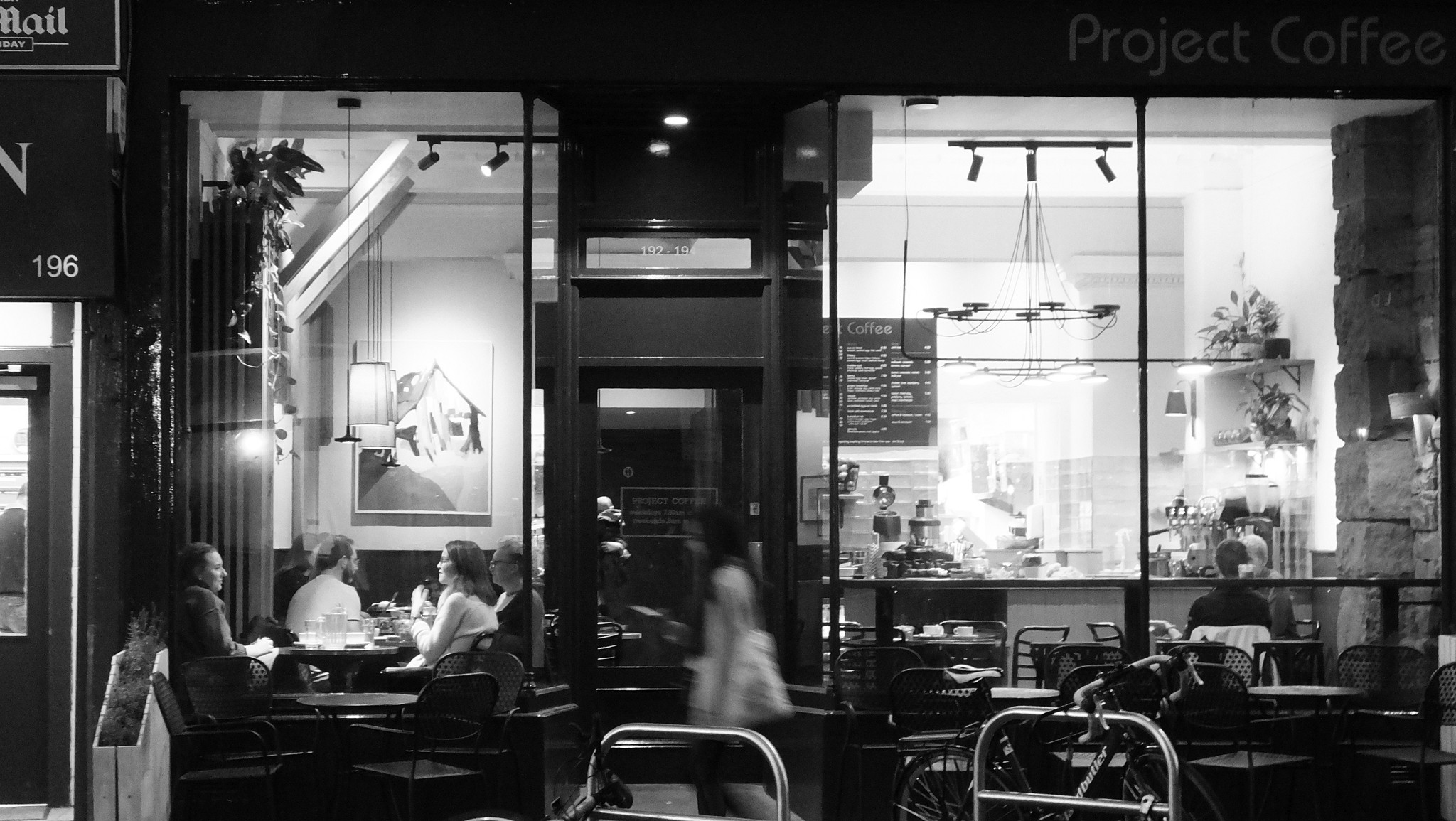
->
[798,474,830,523]
[354,339,493,515]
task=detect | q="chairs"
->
[153,619,1456,821]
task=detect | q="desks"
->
[936,687,1060,817]
[1246,685,1364,820]
[274,646,399,694]
[893,632,997,683]
[297,692,418,820]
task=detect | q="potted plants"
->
[1194,288,1283,366]
[1242,374,1308,446]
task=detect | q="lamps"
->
[332,98,400,468]
[1165,378,1196,438]
[968,148,984,181]
[919,150,1120,386]
[1094,146,1116,183]
[481,140,510,178]
[418,140,442,172]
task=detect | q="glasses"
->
[350,557,360,565]
[490,560,515,567]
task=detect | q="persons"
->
[395,541,499,669]
[681,503,807,821]
[595,495,632,627]
[1178,539,1272,671]
[284,533,374,650]
[487,537,548,671]
[273,535,333,624]
[167,541,319,721]
[1235,534,1298,641]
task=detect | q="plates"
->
[345,641,371,647]
[293,641,323,647]
[952,634,978,638]
[913,634,947,639]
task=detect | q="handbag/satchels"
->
[683,629,795,737]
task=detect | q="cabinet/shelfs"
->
[1202,360,1316,451]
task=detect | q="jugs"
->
[422,607,438,630]
[316,607,347,650]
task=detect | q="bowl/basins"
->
[839,567,858,576]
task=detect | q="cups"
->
[360,619,375,642]
[923,625,944,635]
[346,632,366,644]
[1239,564,1256,579]
[374,628,380,637]
[304,620,318,650]
[299,632,315,643]
[953,626,973,635]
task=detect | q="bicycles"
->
[892,642,1205,821]
